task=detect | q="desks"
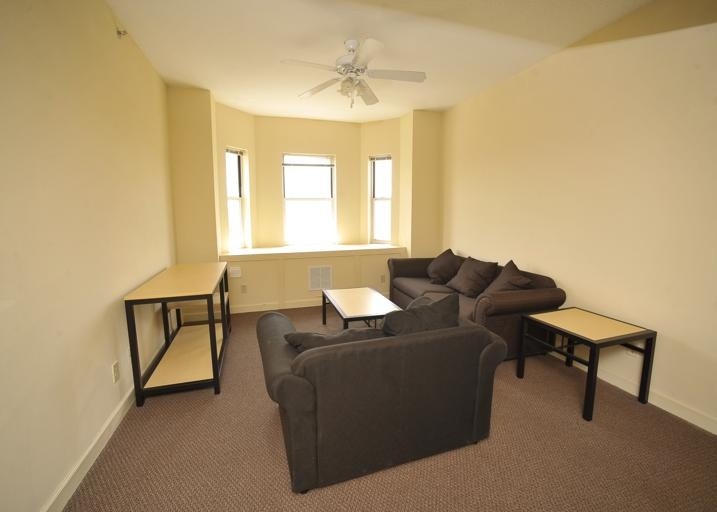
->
[516,306,657,421]
[123,261,232,408]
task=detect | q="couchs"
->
[388,254,566,361]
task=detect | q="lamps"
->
[336,75,365,98]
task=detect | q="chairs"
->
[257,310,508,493]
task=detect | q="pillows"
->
[284,293,459,354]
[426,249,534,299]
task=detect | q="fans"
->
[292,39,425,106]
